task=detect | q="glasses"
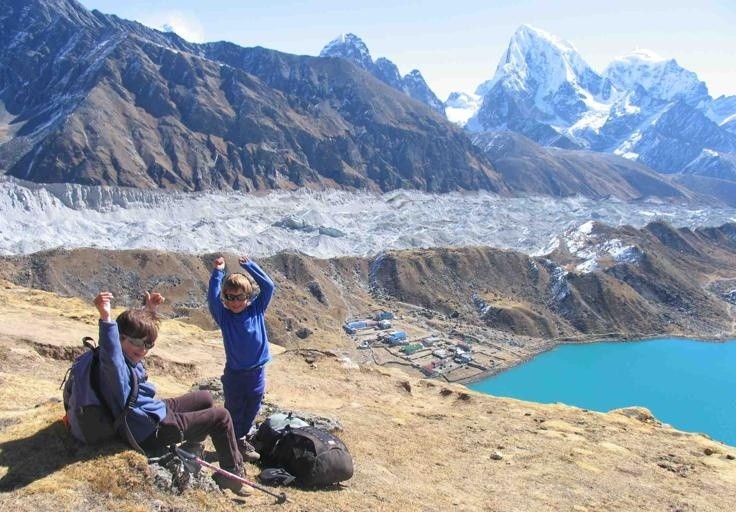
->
[222,291,251,302]
[124,333,155,350]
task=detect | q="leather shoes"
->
[212,470,253,497]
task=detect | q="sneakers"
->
[237,434,262,464]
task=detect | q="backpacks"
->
[61,337,139,445]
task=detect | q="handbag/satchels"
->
[250,410,355,487]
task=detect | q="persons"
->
[93,291,254,496]
[206,254,275,462]
